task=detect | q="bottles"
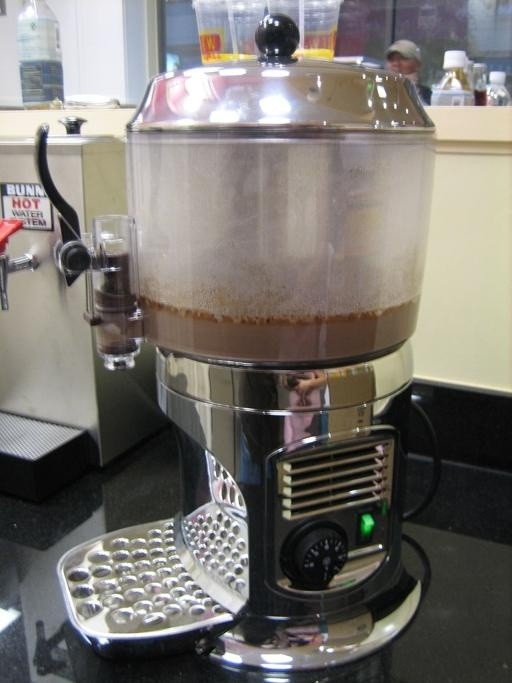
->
[431,50,511,107]
[15,1,65,108]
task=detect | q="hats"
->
[384,39,420,62]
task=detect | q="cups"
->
[191,1,267,68]
[267,2,345,63]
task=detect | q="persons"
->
[234,372,331,485]
[383,39,434,105]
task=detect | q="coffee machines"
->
[33,14,439,676]
